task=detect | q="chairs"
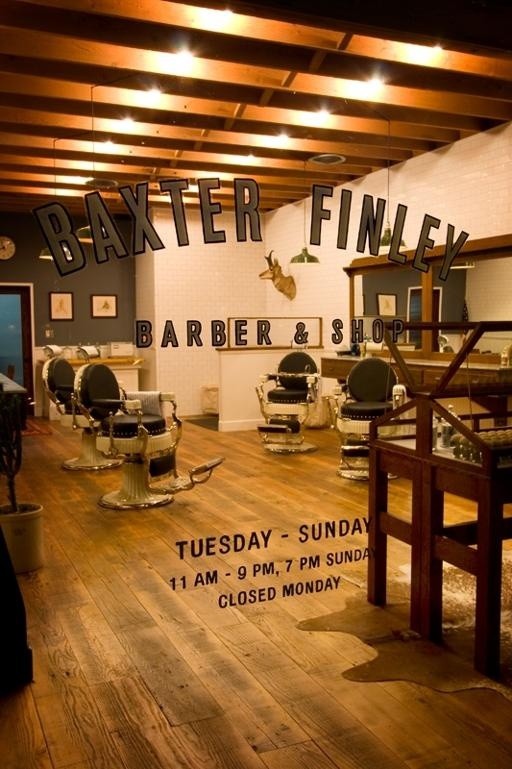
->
[326,357,408,481]
[254,351,320,453]
[42,356,125,471]
[71,364,226,510]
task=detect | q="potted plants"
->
[0,363,46,575]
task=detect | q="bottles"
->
[431,403,460,452]
[500,347,509,367]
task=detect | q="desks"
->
[320,356,512,453]
[0,374,36,698]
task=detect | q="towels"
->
[309,373,319,397]
[124,389,165,417]
[391,384,407,417]
